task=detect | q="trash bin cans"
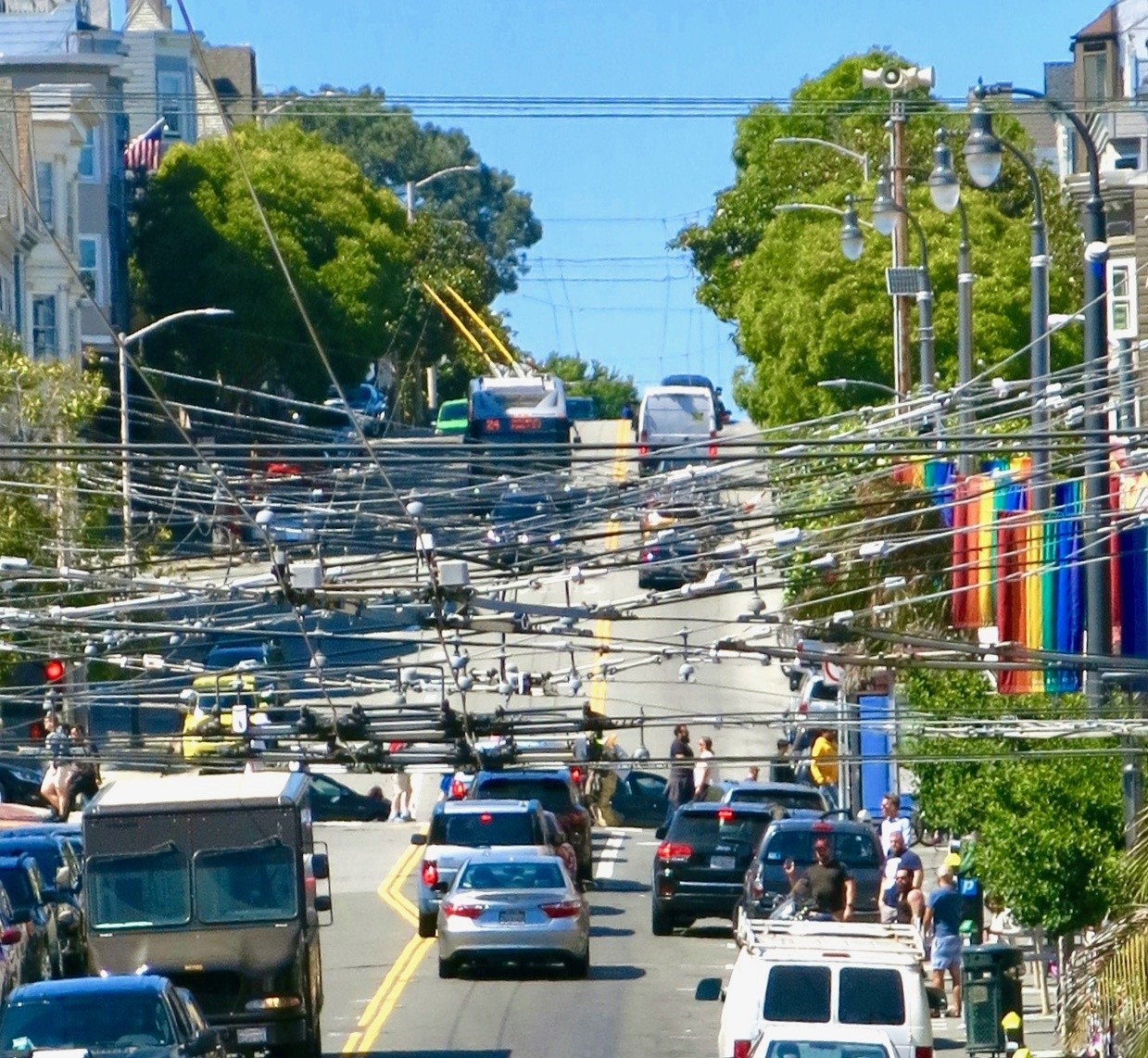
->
[963,942,1025,1058]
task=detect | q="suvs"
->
[610,773,854,937]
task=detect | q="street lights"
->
[118,307,234,599]
[835,81,1105,705]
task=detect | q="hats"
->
[814,837,829,847]
[179,689,196,701]
[937,864,953,877]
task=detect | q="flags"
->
[123,120,166,170]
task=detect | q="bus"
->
[464,373,582,520]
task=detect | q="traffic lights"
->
[46,662,63,679]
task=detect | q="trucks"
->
[42,772,333,1058]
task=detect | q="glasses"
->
[890,840,899,843]
[881,804,888,808]
[699,743,705,746]
[896,876,907,880]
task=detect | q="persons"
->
[40,714,101,822]
[984,891,1026,976]
[811,729,838,812]
[392,742,416,822]
[783,836,856,922]
[923,864,961,1017]
[656,724,695,840]
[770,739,790,782]
[693,736,719,802]
[744,766,759,781]
[878,792,928,978]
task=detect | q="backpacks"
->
[596,753,610,778]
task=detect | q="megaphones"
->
[860,67,937,90]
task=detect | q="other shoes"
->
[44,815,66,823]
[390,812,416,823]
[945,1009,961,1018]
[931,1011,940,1018]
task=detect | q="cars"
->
[733,819,887,946]
[429,855,599,978]
[288,382,468,465]
[779,636,840,749]
[462,768,597,882]
[746,1025,902,1058]
[308,772,380,822]
[0,975,226,1058]
[487,492,713,590]
[0,759,85,1010]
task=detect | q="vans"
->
[634,385,718,478]
[411,799,549,937]
[181,642,275,764]
[694,919,932,1058]
